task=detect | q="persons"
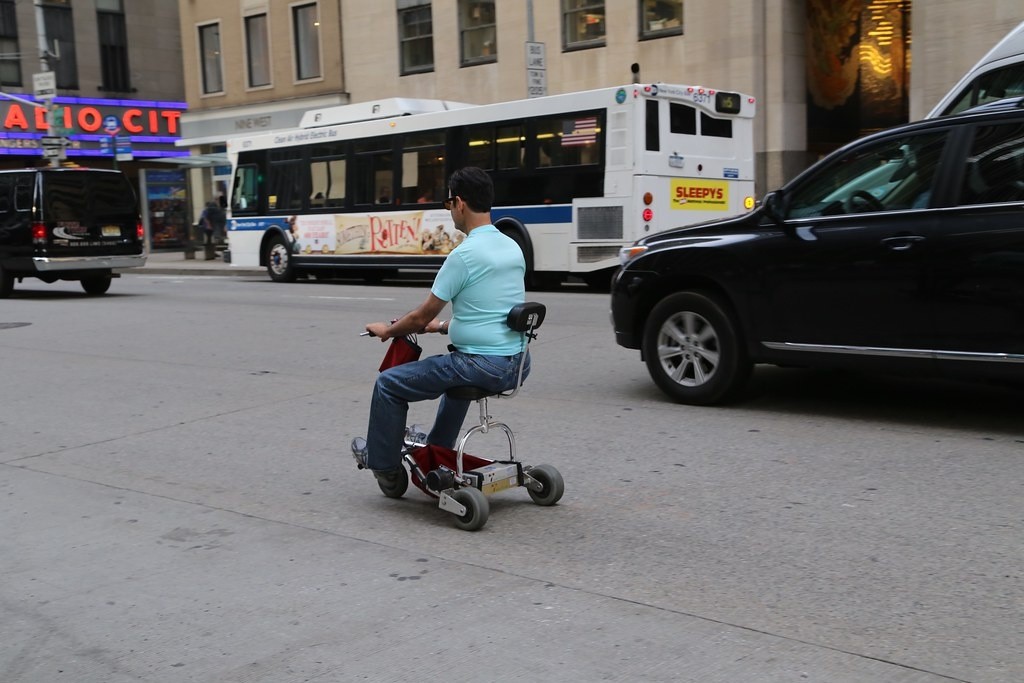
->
[911,142,945,209]
[199,202,225,256]
[350,165,531,478]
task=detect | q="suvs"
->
[606,95,1024,406]
[0,169,146,295]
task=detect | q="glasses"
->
[442,196,460,211]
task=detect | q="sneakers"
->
[406,425,428,446]
[352,434,400,487]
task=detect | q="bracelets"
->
[439,321,448,335]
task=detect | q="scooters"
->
[357,302,565,531]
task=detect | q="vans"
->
[803,15,1024,216]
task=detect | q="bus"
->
[226,83,755,282]
